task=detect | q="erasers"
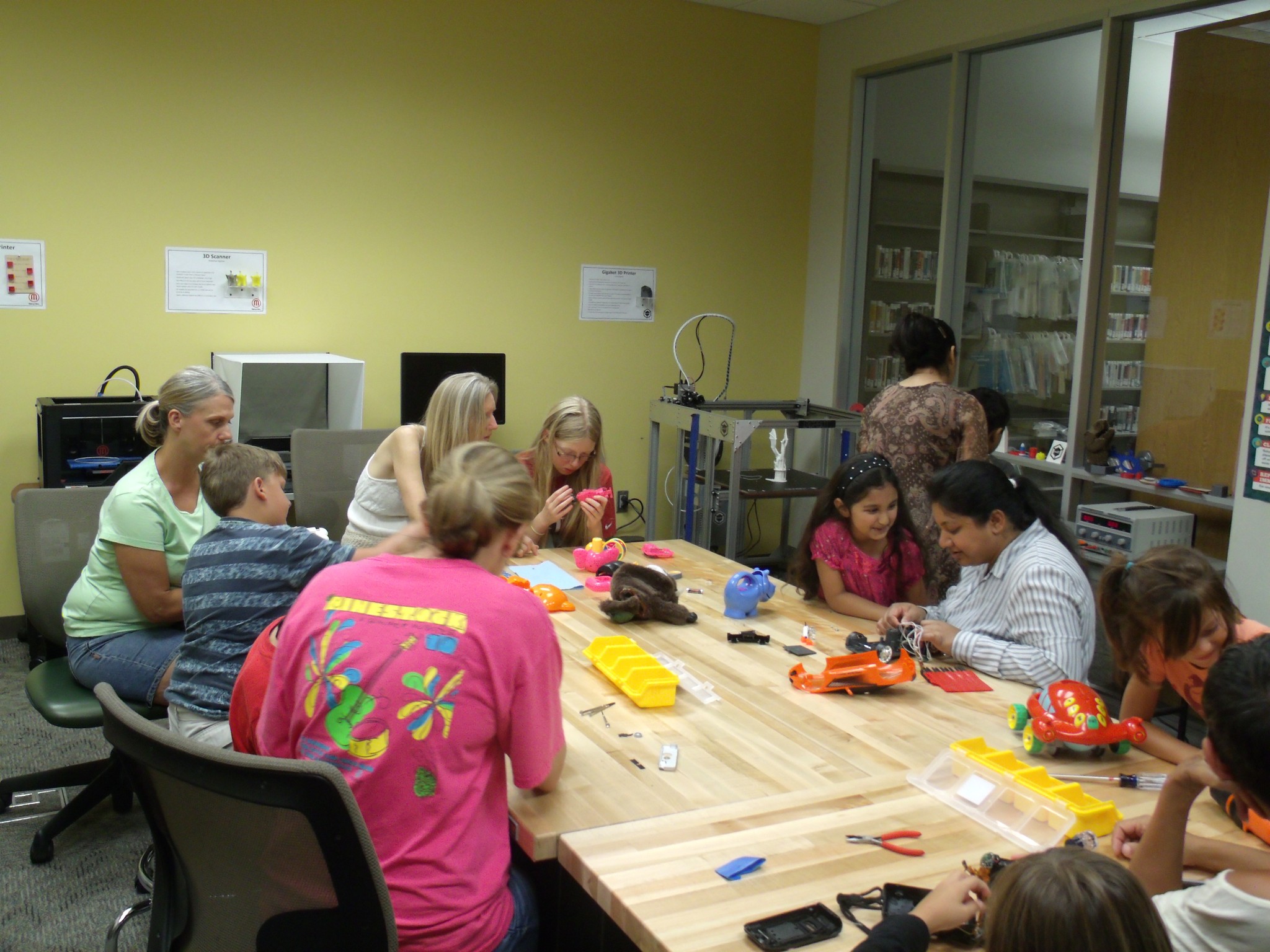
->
[685,587,704,594]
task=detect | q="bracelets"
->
[530,525,546,535]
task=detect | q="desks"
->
[558,721,1269,952]
[505,541,1047,866]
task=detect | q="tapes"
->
[667,569,682,581]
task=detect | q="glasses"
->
[553,440,597,461]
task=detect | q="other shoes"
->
[134,843,154,894]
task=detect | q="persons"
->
[255,439,568,952]
[845,847,1175,952]
[230,614,287,755]
[874,457,1096,691]
[512,394,619,550]
[339,371,541,559]
[781,448,929,623]
[165,442,433,755]
[1108,631,1270,951]
[1097,540,1270,768]
[62,365,237,709]
[966,386,1017,479]
[856,312,991,607]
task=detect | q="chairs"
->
[289,426,392,545]
[1,482,167,871]
[88,679,402,952]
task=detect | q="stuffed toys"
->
[598,563,698,626]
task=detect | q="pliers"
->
[845,830,924,858]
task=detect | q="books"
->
[863,243,1156,434]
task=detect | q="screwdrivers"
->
[1044,773,1169,792]
